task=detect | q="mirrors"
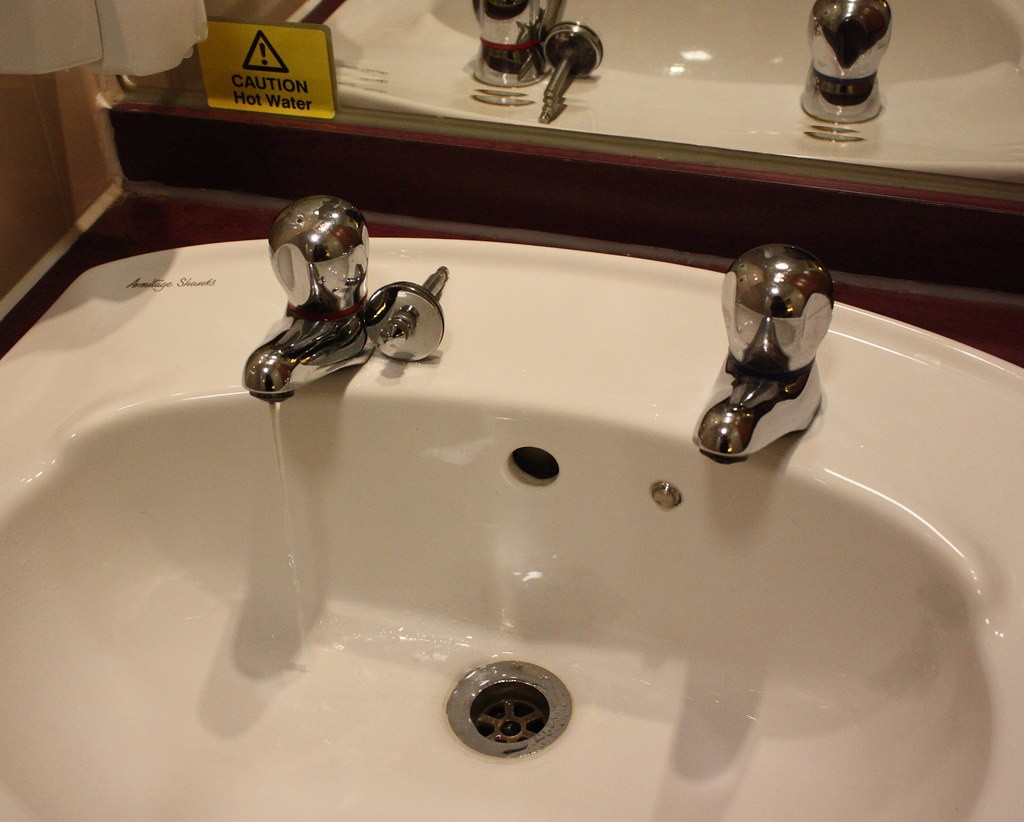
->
[111,0,1024,184]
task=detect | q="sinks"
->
[0,389,1001,818]
[431,0,1024,89]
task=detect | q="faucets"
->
[471,2,566,87]
[692,242,834,465]
[240,193,372,407]
[799,0,894,125]
